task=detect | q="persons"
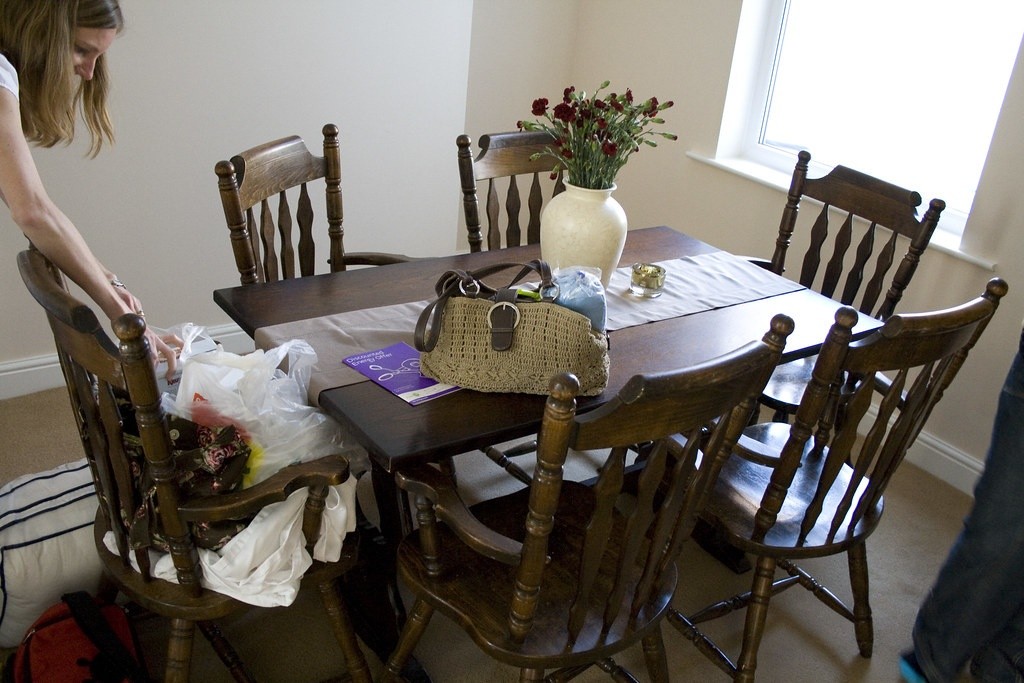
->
[0,0,186,383]
[898,320,1024,683]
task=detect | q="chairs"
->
[379,313,795,683]
[456,125,568,256]
[695,149,946,460]
[214,124,410,287]
[635,276,1009,683]
[16,229,378,683]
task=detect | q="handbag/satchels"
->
[148,321,373,490]
[414,259,609,397]
[1,589,147,683]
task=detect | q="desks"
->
[214,225,890,682]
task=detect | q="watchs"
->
[111,280,127,289]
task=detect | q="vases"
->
[540,175,628,291]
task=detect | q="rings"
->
[136,310,146,317]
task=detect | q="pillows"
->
[0,455,104,651]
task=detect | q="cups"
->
[628,262,666,298]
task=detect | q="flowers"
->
[516,79,678,190]
